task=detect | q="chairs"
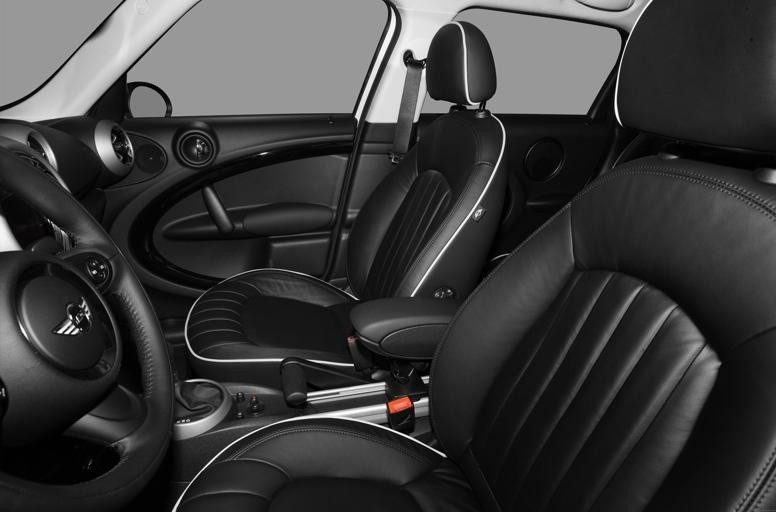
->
[183,20,517,382]
[170,0,776,512]
